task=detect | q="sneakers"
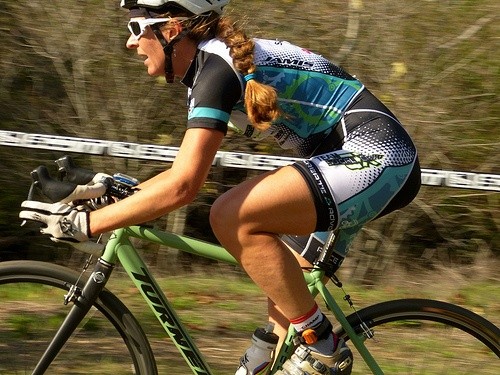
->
[271,339,354,375]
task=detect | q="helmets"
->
[119,0,229,17]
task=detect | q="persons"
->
[19,0,422,375]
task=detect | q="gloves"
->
[18,199,91,245]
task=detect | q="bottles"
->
[235,322,280,375]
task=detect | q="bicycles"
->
[0,157,500,375]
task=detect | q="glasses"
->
[127,17,184,39]
